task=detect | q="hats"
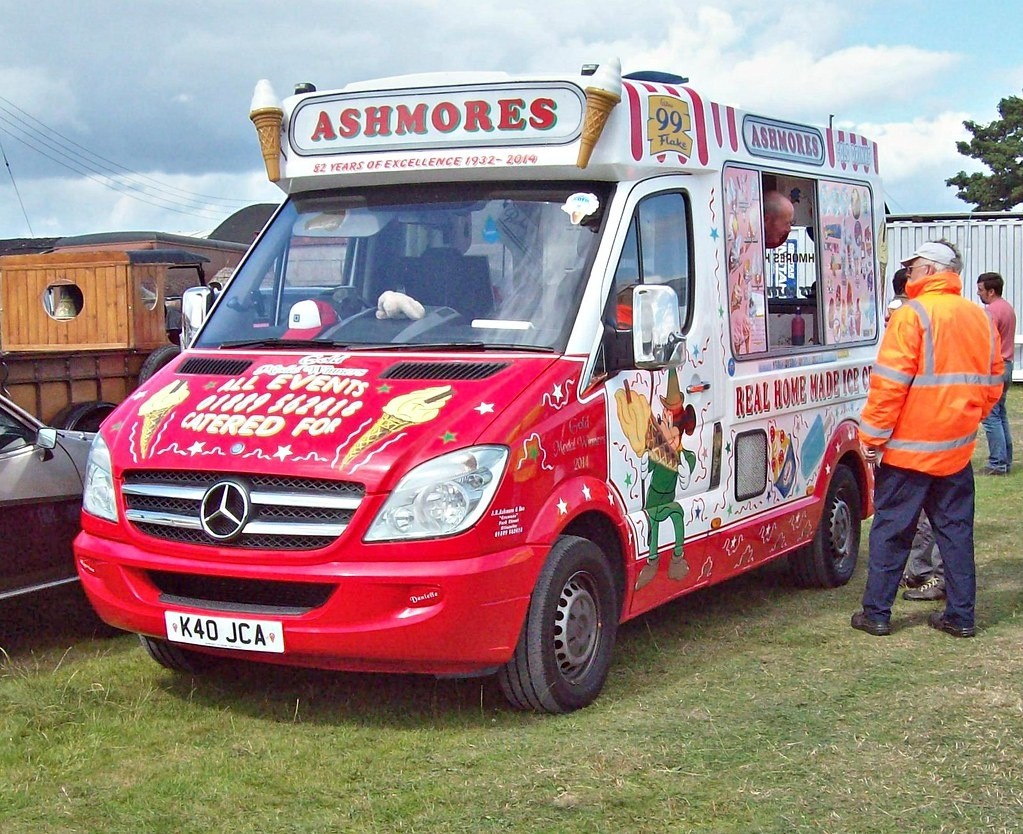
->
[283,298,336,340]
[900,242,959,267]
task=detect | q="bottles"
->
[791,307,805,345]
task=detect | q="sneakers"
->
[928,612,975,638]
[851,610,892,635]
[899,573,921,588]
[902,576,947,601]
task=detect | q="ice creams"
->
[876,217,889,315]
[340,384,454,470]
[576,55,622,170]
[249,79,285,182]
[136,378,189,459]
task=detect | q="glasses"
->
[906,265,926,274]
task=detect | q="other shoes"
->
[977,467,1005,476]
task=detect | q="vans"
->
[73,51,889,715]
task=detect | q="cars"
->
[1,395,131,640]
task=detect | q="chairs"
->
[401,245,485,317]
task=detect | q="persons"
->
[850,242,1006,637]
[762,189,794,244]
[884,239,1017,601]
